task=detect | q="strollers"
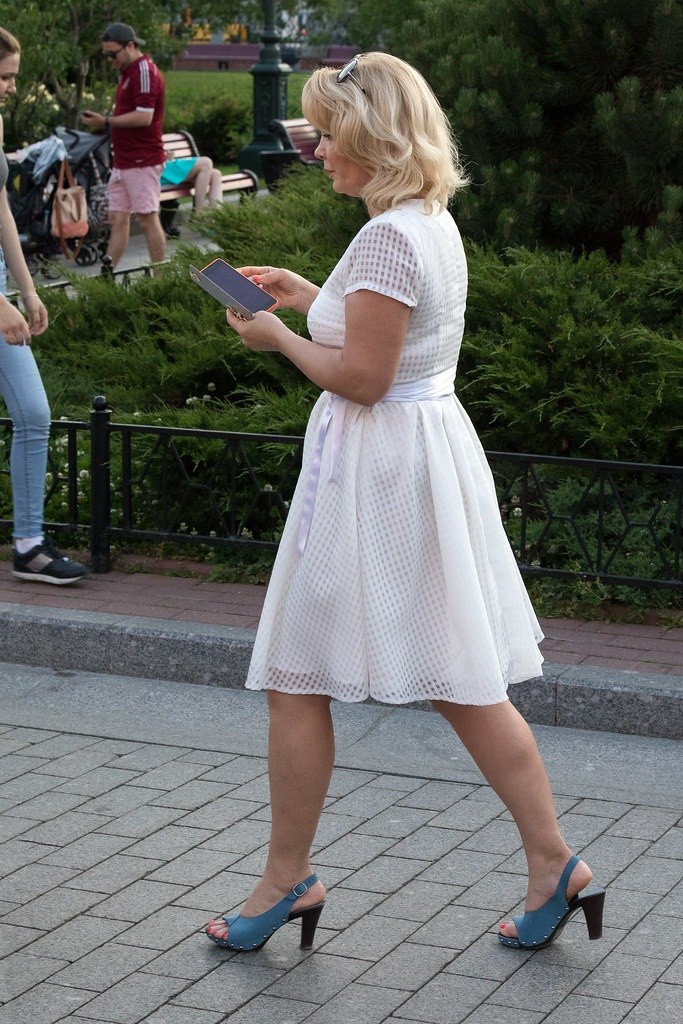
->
[3,106,108,282]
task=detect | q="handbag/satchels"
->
[47,157,89,260]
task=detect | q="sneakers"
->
[12,539,86,585]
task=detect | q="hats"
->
[100,22,147,45]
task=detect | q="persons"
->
[80,23,166,277]
[160,151,223,208]
[0,26,86,584]
[205,52,607,953]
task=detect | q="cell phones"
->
[199,258,279,313]
[82,112,92,118]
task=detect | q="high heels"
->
[206,874,326,951]
[498,855,606,951]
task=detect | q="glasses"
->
[103,42,127,59]
[336,53,366,95]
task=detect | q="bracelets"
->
[23,295,38,300]
[105,117,110,129]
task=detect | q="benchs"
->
[110,131,260,236]
[268,118,323,168]
